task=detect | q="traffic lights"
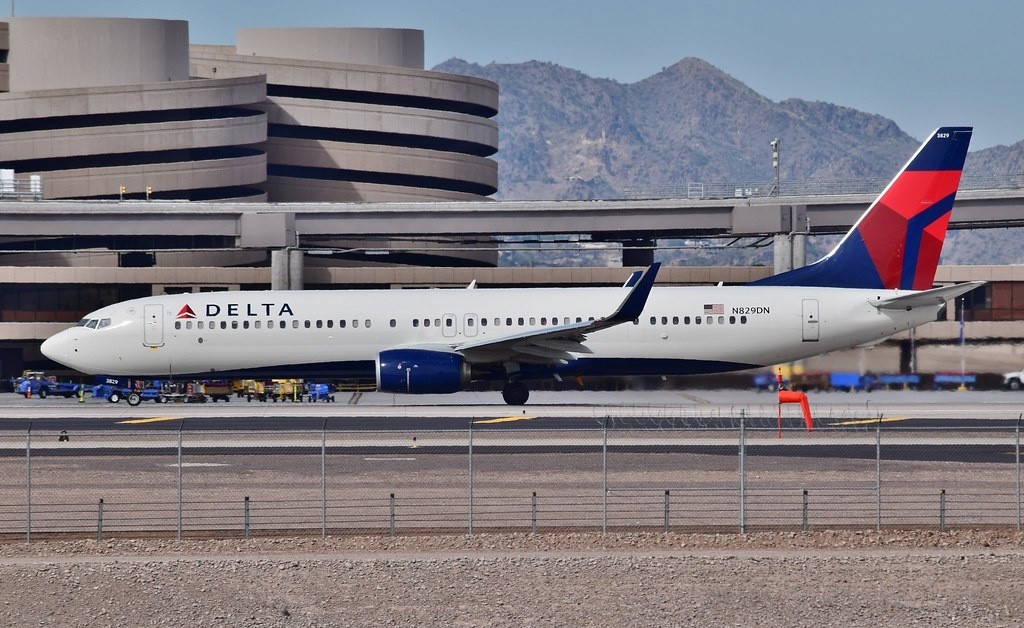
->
[146,186,153,194]
[120,185,127,196]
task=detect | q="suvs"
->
[1000,368,1024,391]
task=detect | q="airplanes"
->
[38,127,991,405]
